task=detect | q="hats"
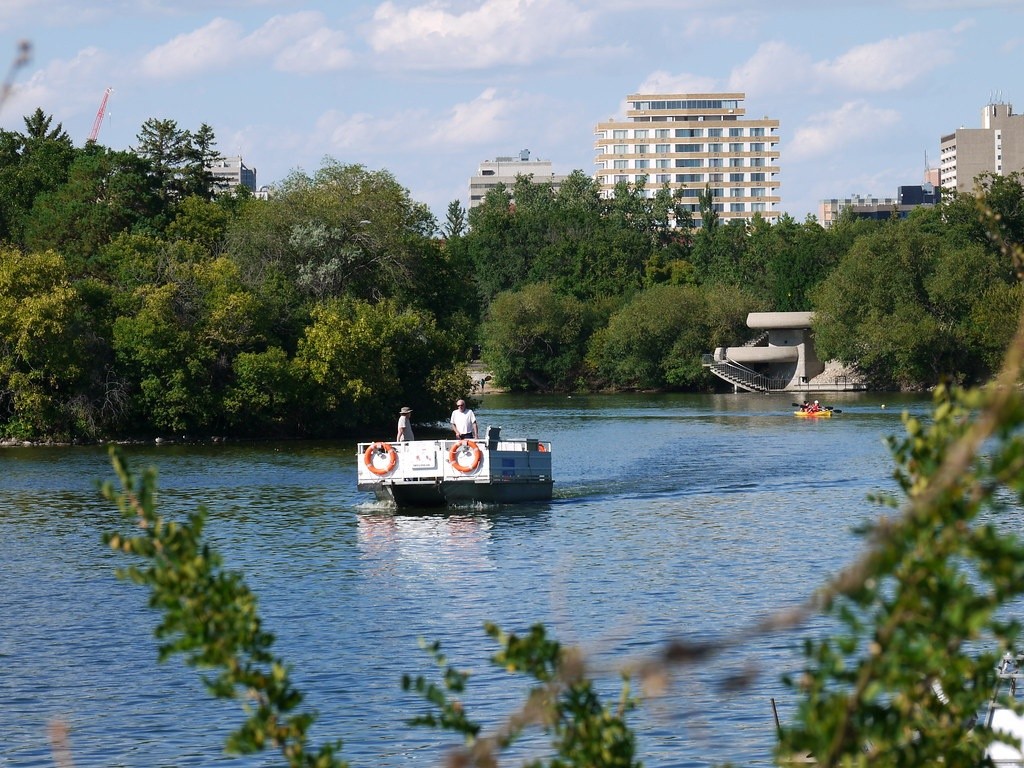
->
[399,407,412,413]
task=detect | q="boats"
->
[794,410,833,418]
[355,426,555,508]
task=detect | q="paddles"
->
[792,402,834,410]
[800,405,842,413]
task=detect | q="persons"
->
[450,400,479,439]
[800,400,826,412]
[397,407,414,442]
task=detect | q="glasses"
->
[458,404,464,407]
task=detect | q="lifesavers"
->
[364,441,399,475]
[448,440,482,473]
